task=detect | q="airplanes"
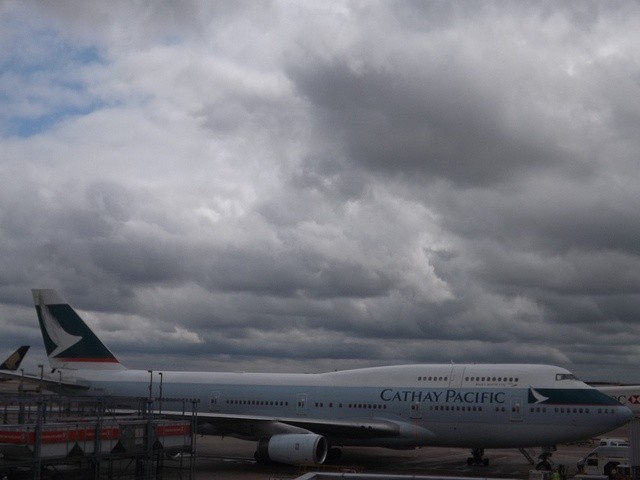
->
[1,289,632,464]
